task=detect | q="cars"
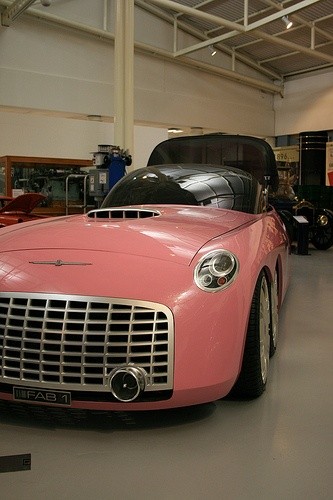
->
[0,193,55,227]
[266,182,333,250]
[0,132,289,411]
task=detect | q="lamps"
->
[281,15,293,29]
[207,45,216,56]
